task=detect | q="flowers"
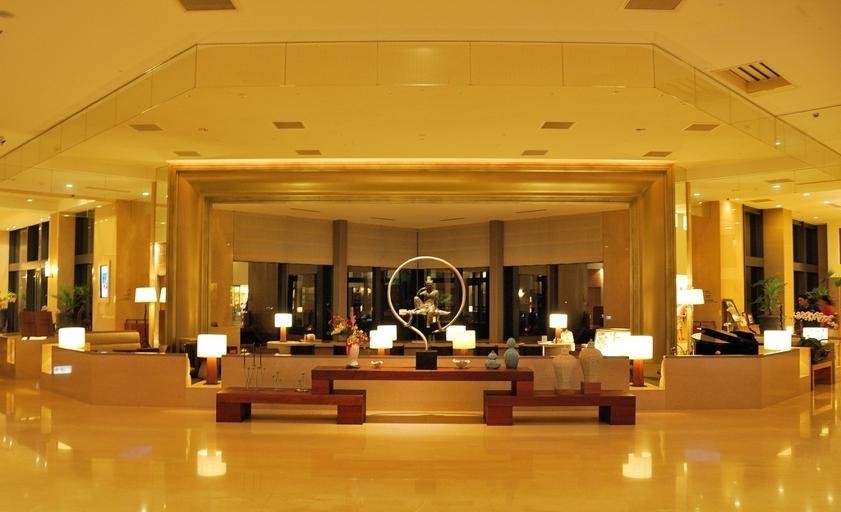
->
[330,307,368,352]
[793,311,838,330]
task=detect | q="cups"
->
[541,336,549,342]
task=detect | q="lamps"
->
[446,325,475,361]
[369,325,397,355]
[58,327,85,350]
[275,313,293,342]
[594,328,654,385]
[134,288,166,347]
[682,289,705,306]
[548,313,569,342]
[763,329,791,351]
[802,327,829,341]
[197,333,227,385]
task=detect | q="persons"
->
[816,295,838,328]
[797,294,821,328]
[414,276,442,328]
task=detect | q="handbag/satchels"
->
[796,337,832,364]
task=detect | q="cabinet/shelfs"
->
[216,366,636,426]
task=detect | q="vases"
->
[344,342,359,366]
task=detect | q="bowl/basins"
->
[368,360,383,369]
[451,359,470,368]
